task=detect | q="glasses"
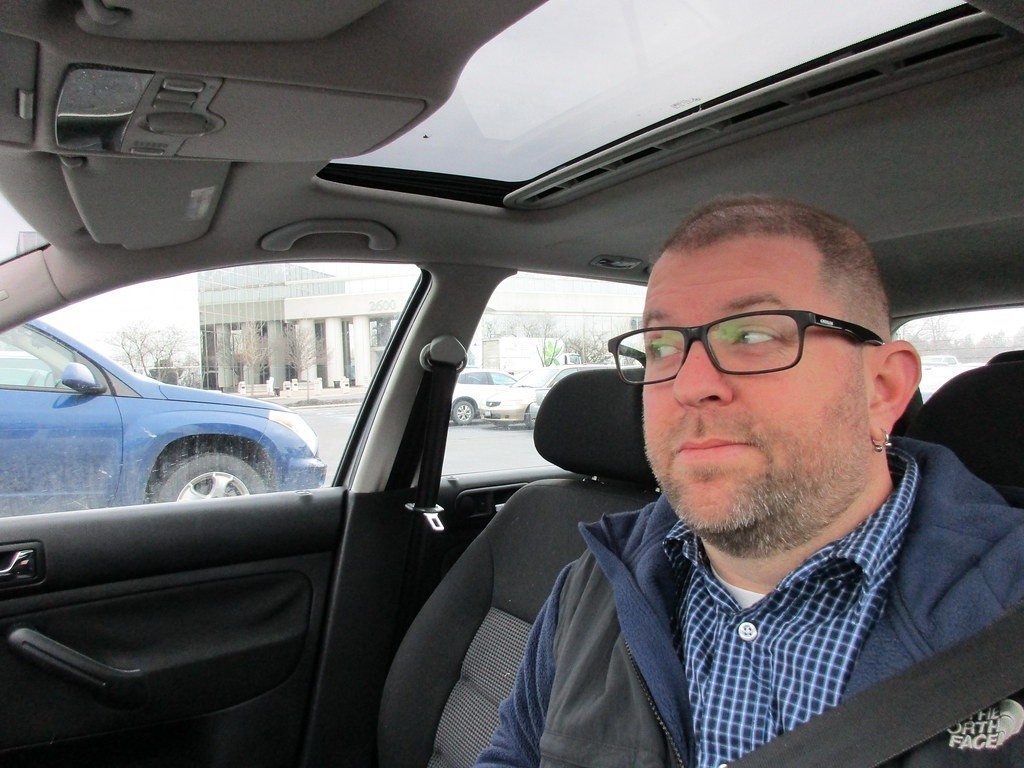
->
[606,309,885,386]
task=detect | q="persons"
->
[474,201,1024,768]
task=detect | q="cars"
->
[1,318,326,519]
[480,364,617,430]
[450,369,520,426]
[921,355,960,373]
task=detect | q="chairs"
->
[903,359,1024,488]
[378,368,660,768]
